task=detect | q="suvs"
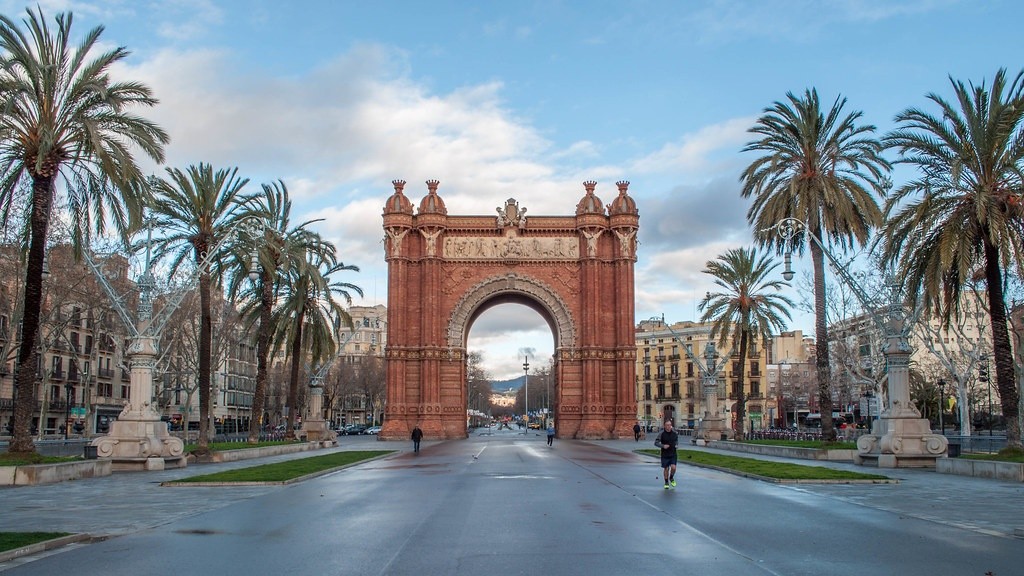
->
[531,422,539,430]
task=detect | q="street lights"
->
[649,310,759,441]
[938,377,946,432]
[978,355,992,437]
[864,392,872,433]
[545,374,549,430]
[777,216,948,468]
[469,380,485,426]
[21,190,267,464]
[64,383,72,439]
[541,379,544,431]
[272,315,376,440]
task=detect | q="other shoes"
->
[670,480,676,487]
[664,482,669,489]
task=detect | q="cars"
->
[335,424,381,434]
[528,423,532,428]
[484,423,496,427]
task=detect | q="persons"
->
[654,421,678,490]
[547,422,555,447]
[633,422,640,442]
[411,424,423,453]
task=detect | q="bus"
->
[804,412,855,432]
